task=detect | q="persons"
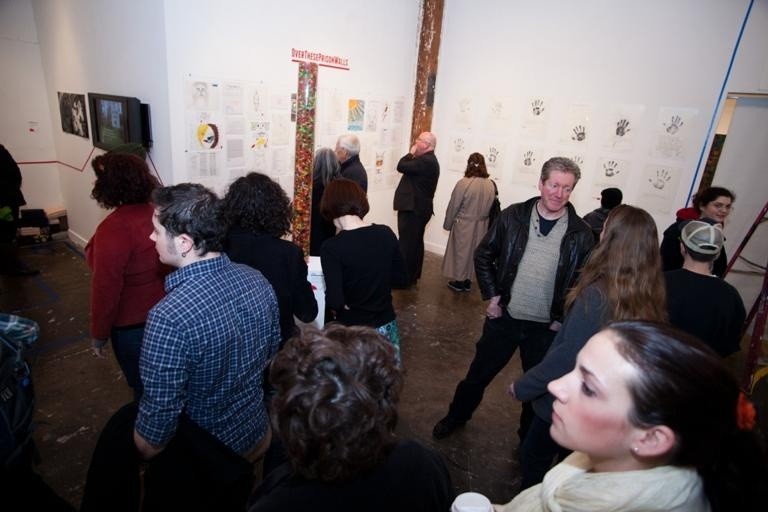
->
[318,178,410,370]
[211,172,318,411]
[432,157,594,459]
[485,321,738,512]
[83,151,177,403]
[128,182,280,511]
[392,131,439,284]
[580,187,748,364]
[308,133,368,258]
[504,204,667,496]
[236,323,454,510]
[442,153,498,292]
[0,144,26,269]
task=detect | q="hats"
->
[681,219,723,255]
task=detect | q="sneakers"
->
[447,280,463,292]
[463,281,472,292]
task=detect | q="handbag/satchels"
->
[1,332,36,463]
[487,198,502,233]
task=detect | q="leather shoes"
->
[432,413,468,440]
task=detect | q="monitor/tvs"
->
[88,93,150,152]
